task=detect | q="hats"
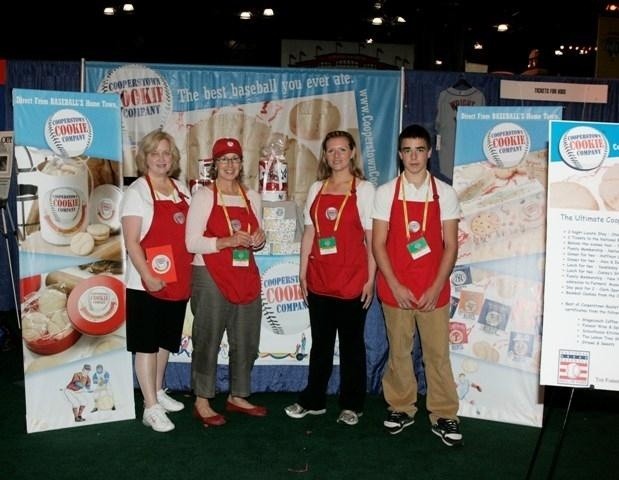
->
[213,139,241,158]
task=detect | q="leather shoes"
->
[225,401,266,416]
[193,405,226,425]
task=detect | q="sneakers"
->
[432,418,464,446]
[142,389,184,432]
[337,410,363,426]
[284,403,326,418]
[384,412,415,434]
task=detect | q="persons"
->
[284,130,378,427]
[177,335,191,359]
[300,333,307,355]
[117,128,193,433]
[371,123,466,448]
[89,364,117,413]
[184,137,268,427]
[66,364,93,423]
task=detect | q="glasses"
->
[216,158,241,163]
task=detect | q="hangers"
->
[439,73,484,97]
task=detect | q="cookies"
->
[186,98,362,206]
[22,288,71,343]
[470,211,501,236]
[70,231,94,257]
[87,224,111,242]
[549,163,619,211]
